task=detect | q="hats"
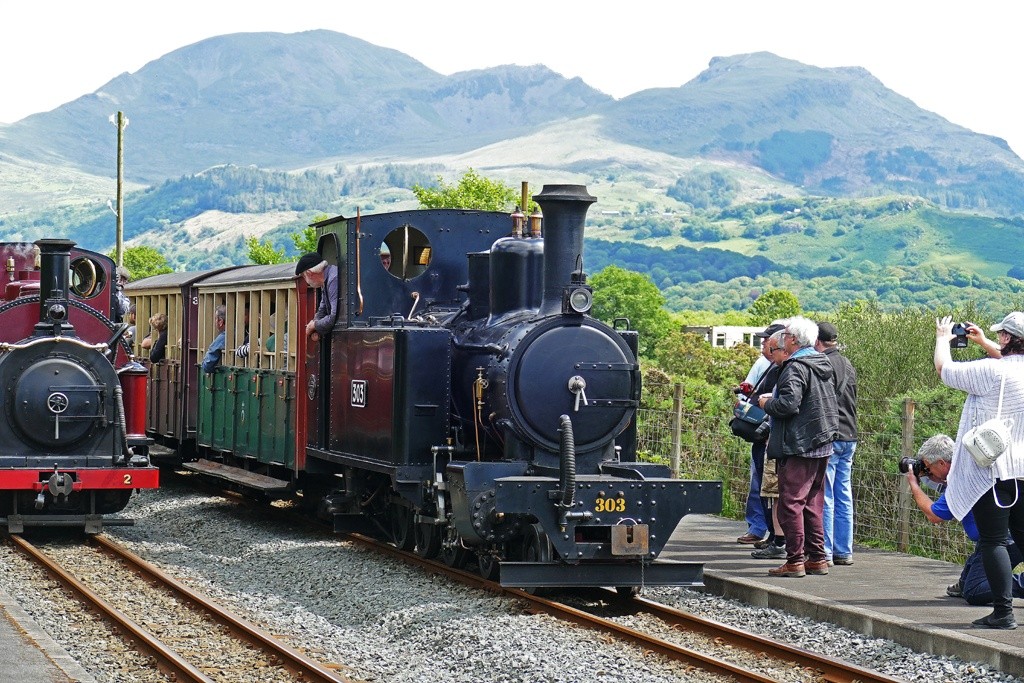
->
[295,252,323,276]
[990,311,1024,340]
[818,322,837,342]
[755,324,786,337]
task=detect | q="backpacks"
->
[962,358,1019,509]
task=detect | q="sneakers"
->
[768,561,806,577]
[804,558,828,575]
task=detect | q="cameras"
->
[899,456,925,476]
[754,413,771,439]
[740,384,751,393]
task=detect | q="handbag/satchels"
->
[730,399,771,441]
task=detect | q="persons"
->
[737,316,858,578]
[202,303,289,373]
[934,311,1024,629]
[141,326,151,349]
[380,241,397,271]
[295,253,338,341]
[149,312,168,363]
[114,267,130,312]
[908,433,1024,605]
[127,304,136,342]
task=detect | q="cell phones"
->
[952,323,970,335]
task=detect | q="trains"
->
[0,238,159,536]
[119,183,728,588]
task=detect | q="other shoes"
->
[972,610,1018,630]
[751,543,787,559]
[946,581,965,598]
[737,532,762,544]
[754,540,774,548]
[834,554,854,565]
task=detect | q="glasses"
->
[780,332,791,338]
[768,346,781,353]
